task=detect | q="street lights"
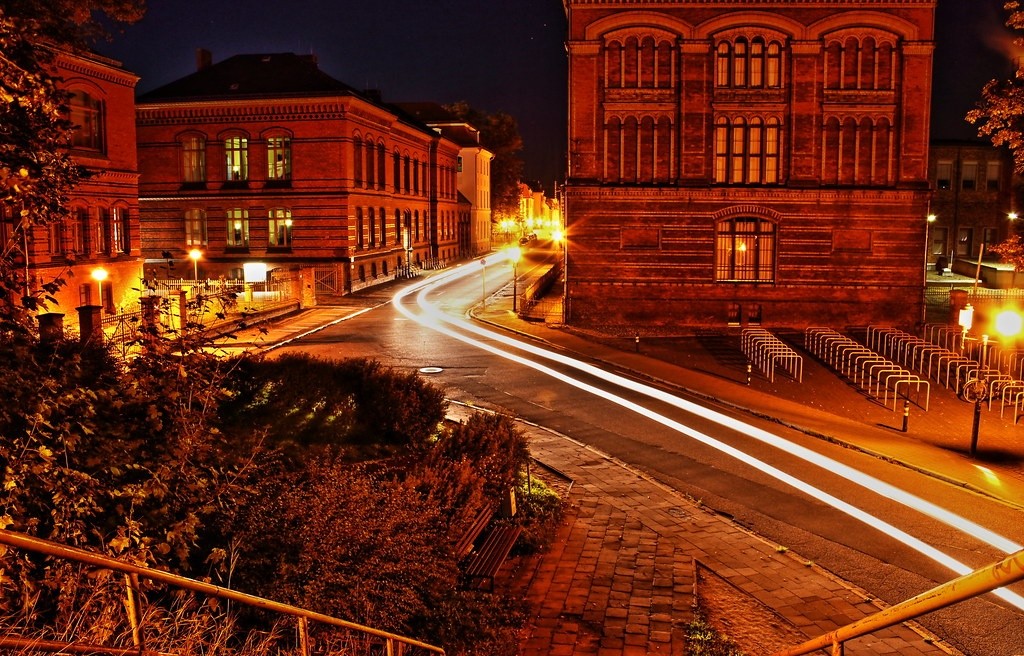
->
[508,247,521,312]
[189,249,201,286]
[92,267,108,318]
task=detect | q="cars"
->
[518,238,529,247]
